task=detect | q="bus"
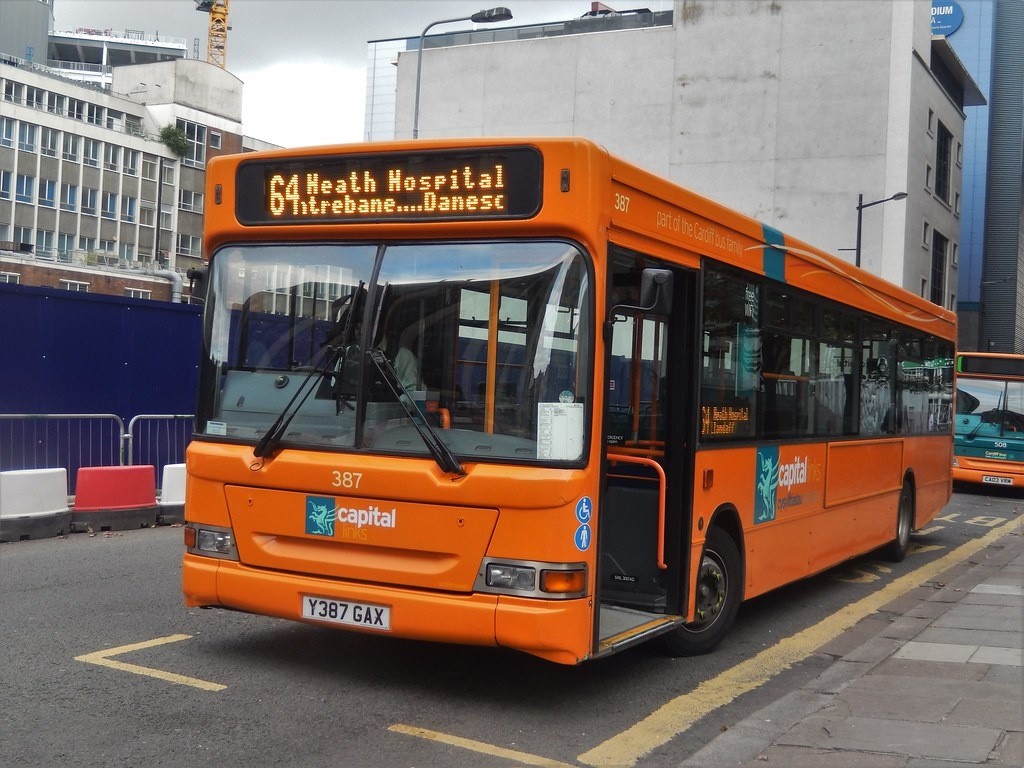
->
[183,137,959,666]
[951,352,1024,488]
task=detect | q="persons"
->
[330,321,419,402]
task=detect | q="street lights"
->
[412,8,513,138]
[855,191,908,267]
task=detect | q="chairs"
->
[479,379,517,425]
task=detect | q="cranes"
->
[193,0,229,70]
